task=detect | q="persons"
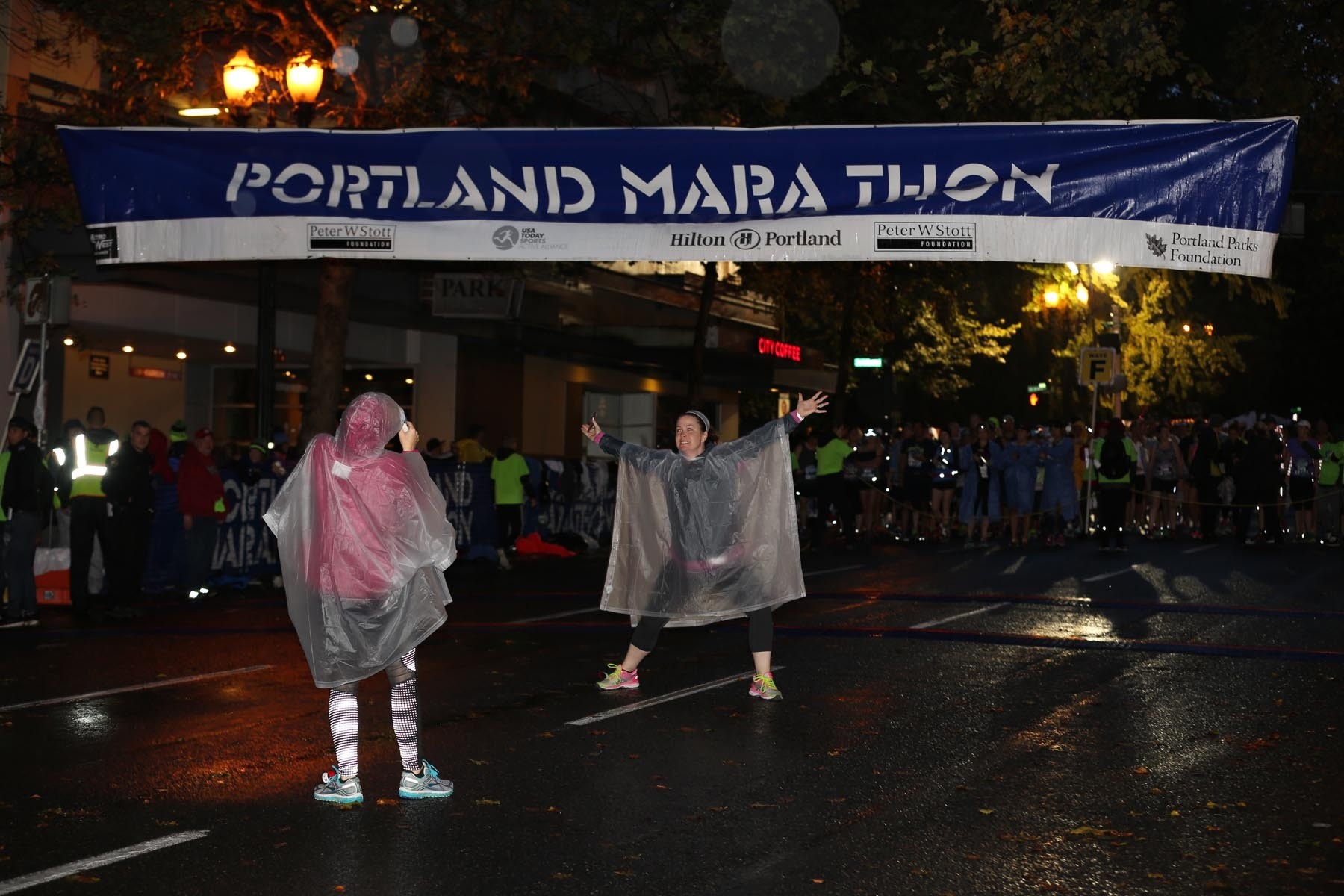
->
[1234,421,1284,548]
[298,392,455,804]
[176,427,230,608]
[489,430,537,570]
[0,415,55,623]
[959,422,1003,548]
[417,425,618,556]
[579,391,831,699]
[57,407,123,624]
[1093,418,1138,552]
[810,412,865,551]
[0,406,309,625]
[701,410,1344,549]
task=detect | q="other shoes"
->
[494,548,512,569]
[102,608,146,621]
[184,570,283,600]
[0,615,39,628]
[799,514,1344,548]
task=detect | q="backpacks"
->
[1098,437,1131,480]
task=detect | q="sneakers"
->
[749,672,783,700]
[399,759,454,799]
[313,765,363,803]
[597,663,640,689]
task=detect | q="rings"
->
[817,404,820,409]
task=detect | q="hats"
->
[1003,415,1014,423]
[1296,420,1311,429]
[194,427,212,439]
[427,437,443,451]
[1013,422,1031,430]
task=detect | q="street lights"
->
[1066,260,1115,347]
[219,44,325,453]
